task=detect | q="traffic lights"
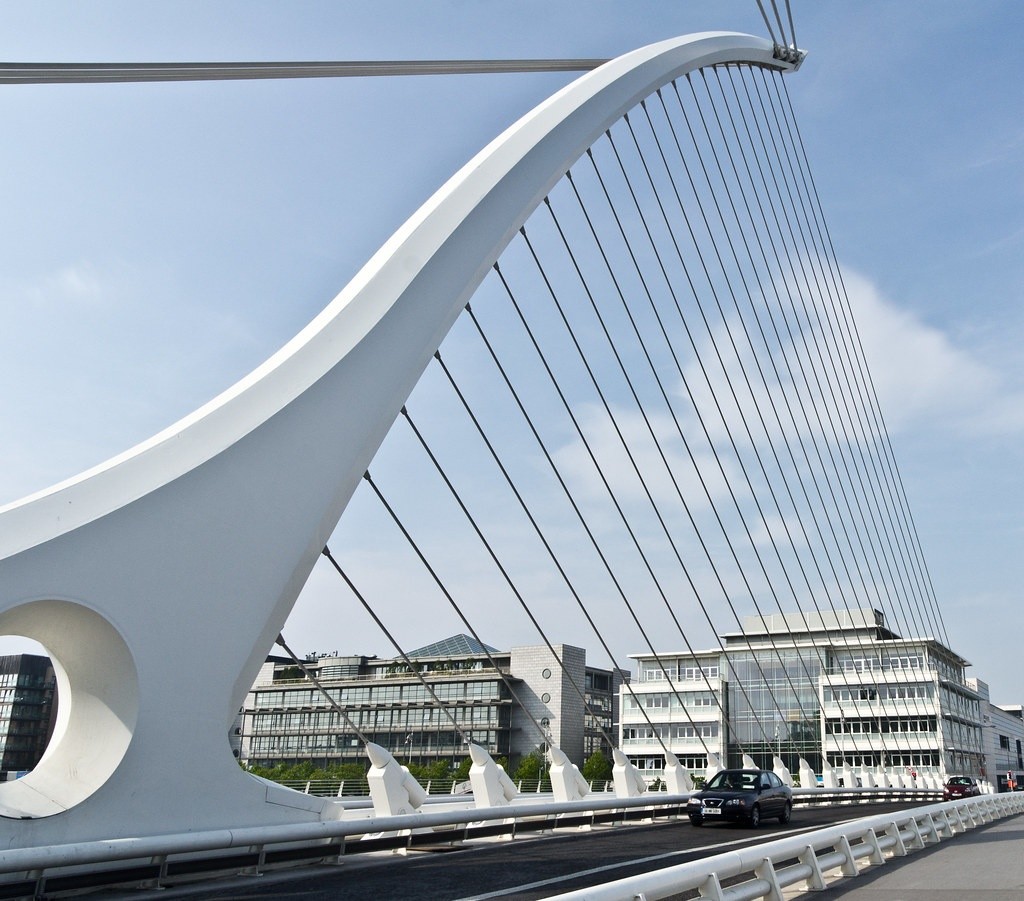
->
[1008,771,1011,779]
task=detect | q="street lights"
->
[405,736,412,764]
[840,710,846,759]
[543,726,551,775]
[775,727,781,760]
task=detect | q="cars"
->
[943,778,980,801]
[687,770,794,823]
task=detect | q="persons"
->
[1007,770,1016,791]
[724,773,739,792]
